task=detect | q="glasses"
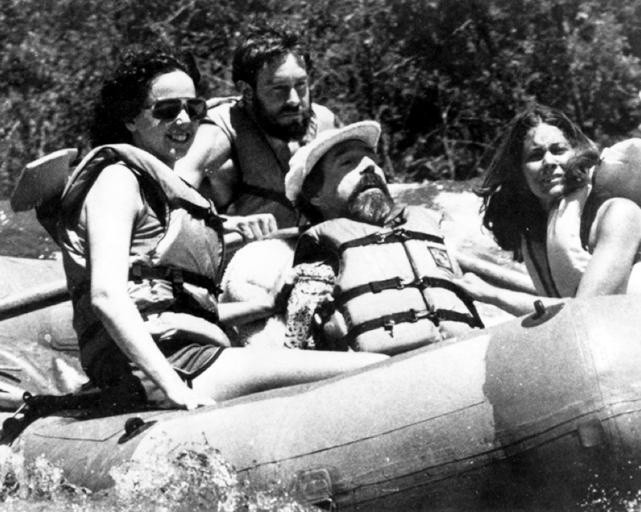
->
[147,95,209,121]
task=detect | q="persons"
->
[273,117,488,357]
[170,26,348,241]
[59,44,393,415]
[446,101,641,319]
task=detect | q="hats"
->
[283,119,382,200]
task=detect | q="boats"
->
[0,256,641,511]
